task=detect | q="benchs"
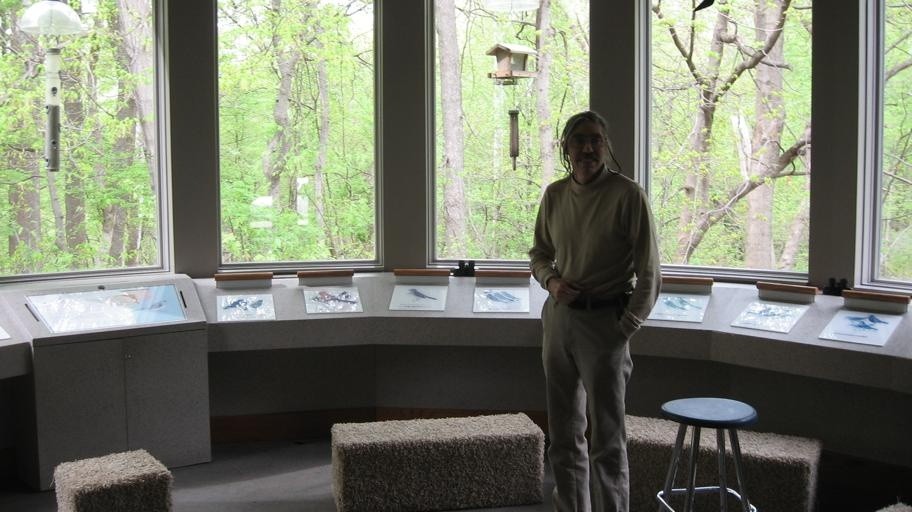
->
[330,413,547,511]
[623,414,821,512]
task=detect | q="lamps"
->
[18,1,86,172]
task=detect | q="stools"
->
[656,396,757,512]
[55,450,171,511]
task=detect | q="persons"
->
[528,111,660,512]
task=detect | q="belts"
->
[567,293,627,310]
